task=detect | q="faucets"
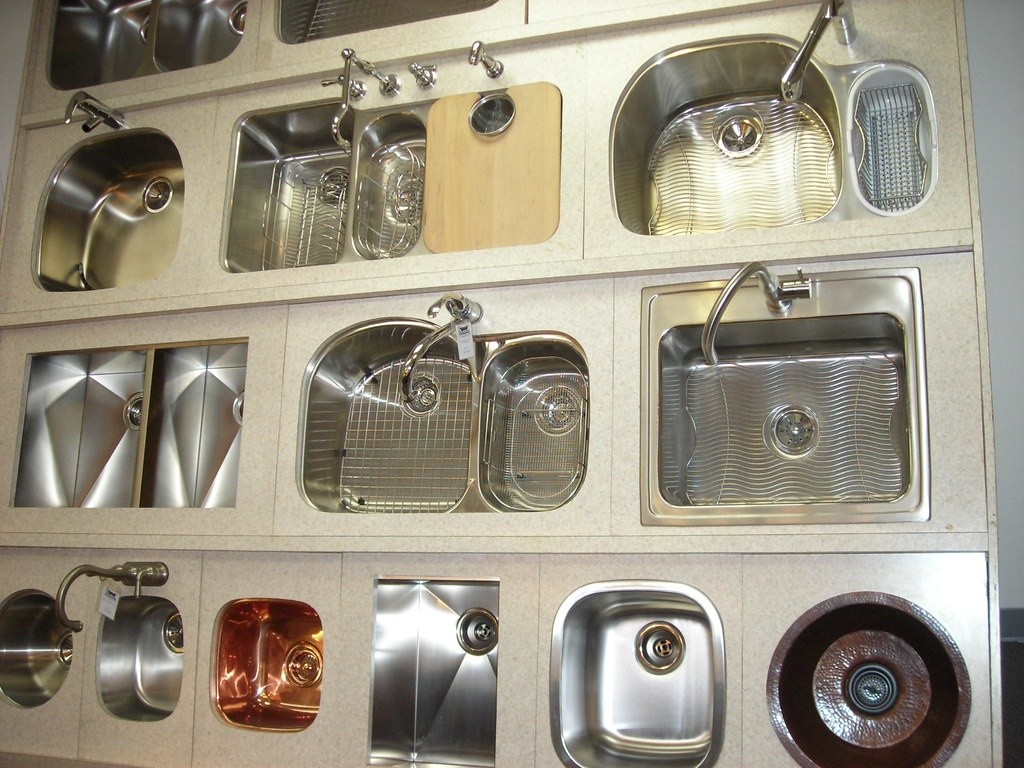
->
[63,91,125,133]
[778,0,857,104]
[701,262,793,365]
[104,0,152,19]
[398,292,484,402]
[468,41,504,79]
[329,48,402,151]
[55,563,138,633]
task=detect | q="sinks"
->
[30,126,186,293]
[218,96,356,274]
[657,310,914,507]
[765,589,972,768]
[608,33,843,236]
[846,60,939,217]
[152,0,248,72]
[209,597,324,733]
[138,334,249,509]
[477,329,590,513]
[274,0,500,45]
[352,97,433,261]
[468,89,516,136]
[95,595,184,722]
[0,588,73,709]
[365,573,501,768]
[549,579,727,768]
[9,343,150,509]
[294,316,474,514]
[45,0,152,91]
